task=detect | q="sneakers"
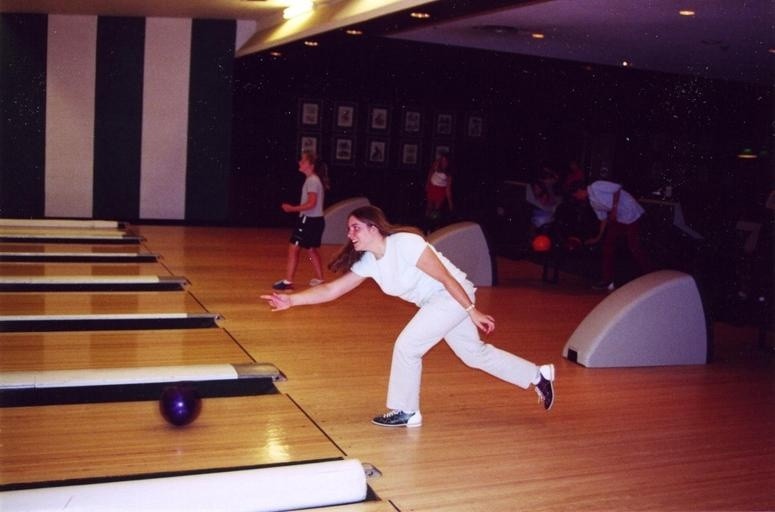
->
[592,280,616,290]
[533,364,555,410]
[272,280,296,291]
[309,279,325,287]
[372,410,423,428]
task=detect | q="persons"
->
[258,205,558,428]
[570,180,648,289]
[421,155,455,234]
[273,150,329,288]
[518,160,608,242]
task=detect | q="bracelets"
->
[465,302,475,314]
